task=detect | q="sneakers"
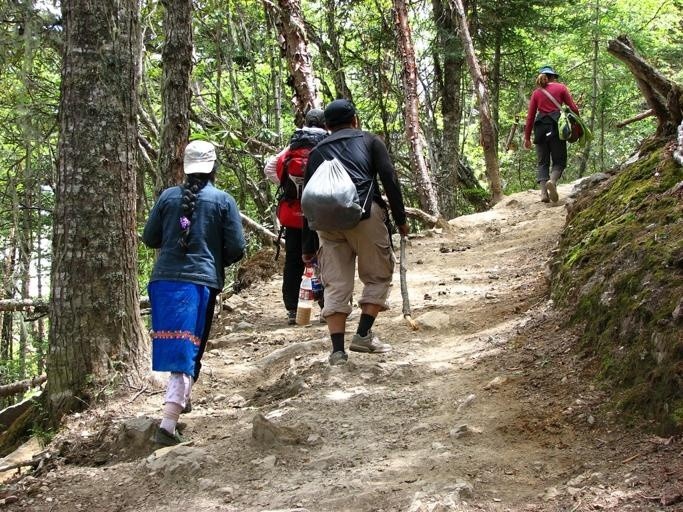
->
[288,313,392,366]
[545,180,559,201]
[541,193,550,203]
[155,428,178,446]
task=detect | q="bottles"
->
[295,258,324,326]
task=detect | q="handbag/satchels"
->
[560,113,583,141]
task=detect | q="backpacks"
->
[277,132,323,228]
[300,140,375,231]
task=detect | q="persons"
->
[302,98,410,366]
[523,66,580,203]
[141,139,245,447]
[264,108,329,325]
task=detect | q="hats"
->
[305,109,324,127]
[183,140,216,174]
[539,66,559,79]
